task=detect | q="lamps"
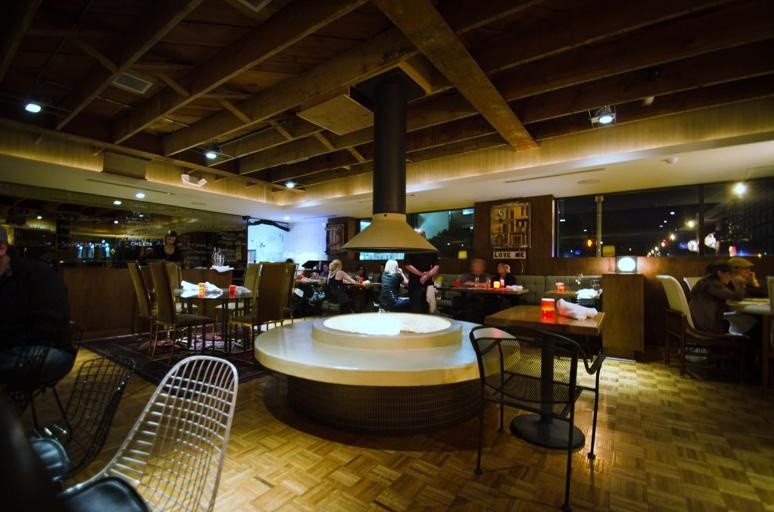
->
[589,103,615,124]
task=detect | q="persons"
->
[463,258,488,282]
[404,229,440,315]
[688,259,760,337]
[491,262,517,288]
[377,263,384,283]
[378,259,409,313]
[326,259,356,314]
[728,257,763,298]
[320,263,328,277]
[354,265,369,281]
[156,229,185,270]
[0,225,74,387]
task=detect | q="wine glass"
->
[591,279,600,293]
[574,271,584,289]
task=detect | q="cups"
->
[541,282,564,320]
[228,284,236,295]
[228,295,235,299]
[198,283,205,298]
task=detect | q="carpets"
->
[71,317,274,398]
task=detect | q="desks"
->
[437,285,529,301]
[299,279,383,289]
[545,289,599,300]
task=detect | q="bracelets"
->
[752,279,757,284]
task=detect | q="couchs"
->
[430,273,601,300]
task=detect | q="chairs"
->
[468,323,605,511]
[64,352,237,511]
[655,271,773,380]
[1,318,83,447]
[33,351,137,481]
[124,257,297,365]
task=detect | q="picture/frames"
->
[488,202,532,250]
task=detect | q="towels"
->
[555,298,598,323]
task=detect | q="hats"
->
[707,258,754,272]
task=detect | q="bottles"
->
[729,244,736,257]
[130,237,152,246]
[74,239,112,259]
[211,247,225,266]
[351,272,380,284]
[475,278,504,289]
